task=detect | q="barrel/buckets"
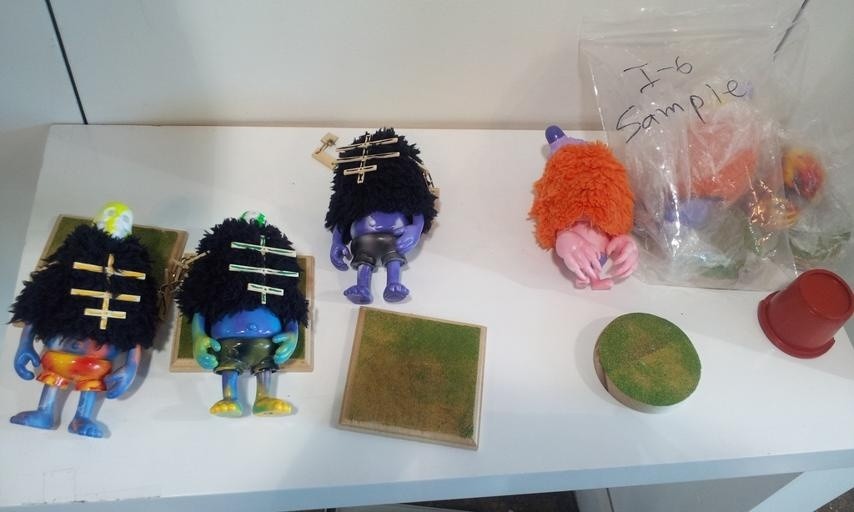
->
[757,268,854,357]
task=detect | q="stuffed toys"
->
[528,125,641,290]
[170,209,312,420]
[4,200,161,436]
[308,124,440,304]
[621,87,849,283]
